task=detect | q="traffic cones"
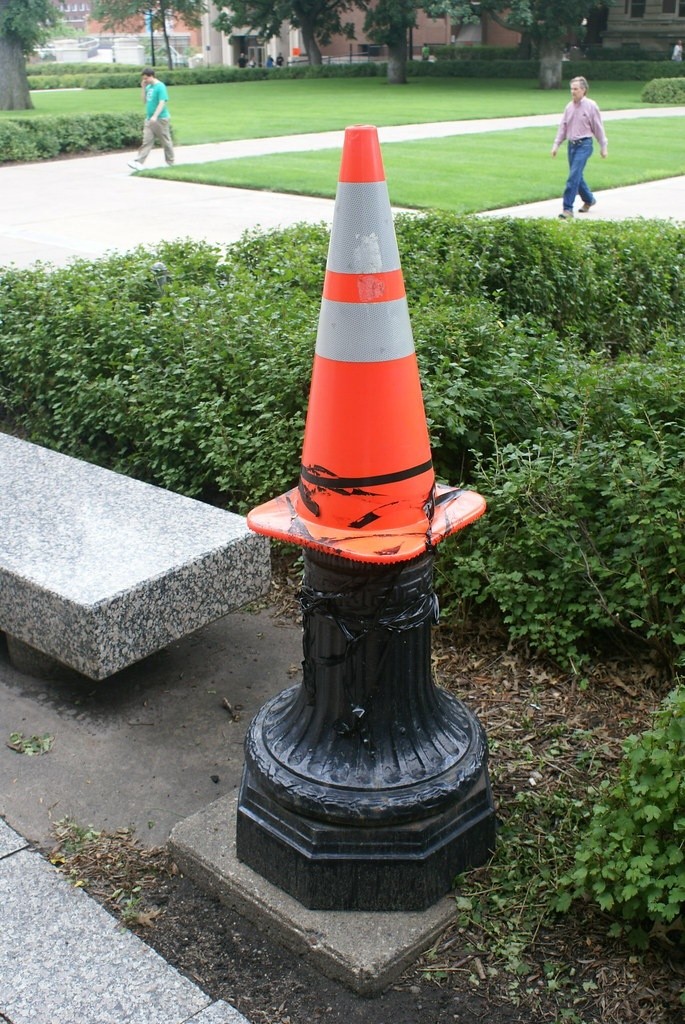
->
[247,123,489,564]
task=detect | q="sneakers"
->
[578,198,596,213]
[559,210,574,218]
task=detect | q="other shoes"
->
[127,161,144,171]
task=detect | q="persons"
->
[249,57,256,68]
[129,69,177,171]
[277,53,284,66]
[238,53,248,67]
[266,55,273,68]
[551,77,609,219]
[421,43,430,61]
[671,40,684,62]
